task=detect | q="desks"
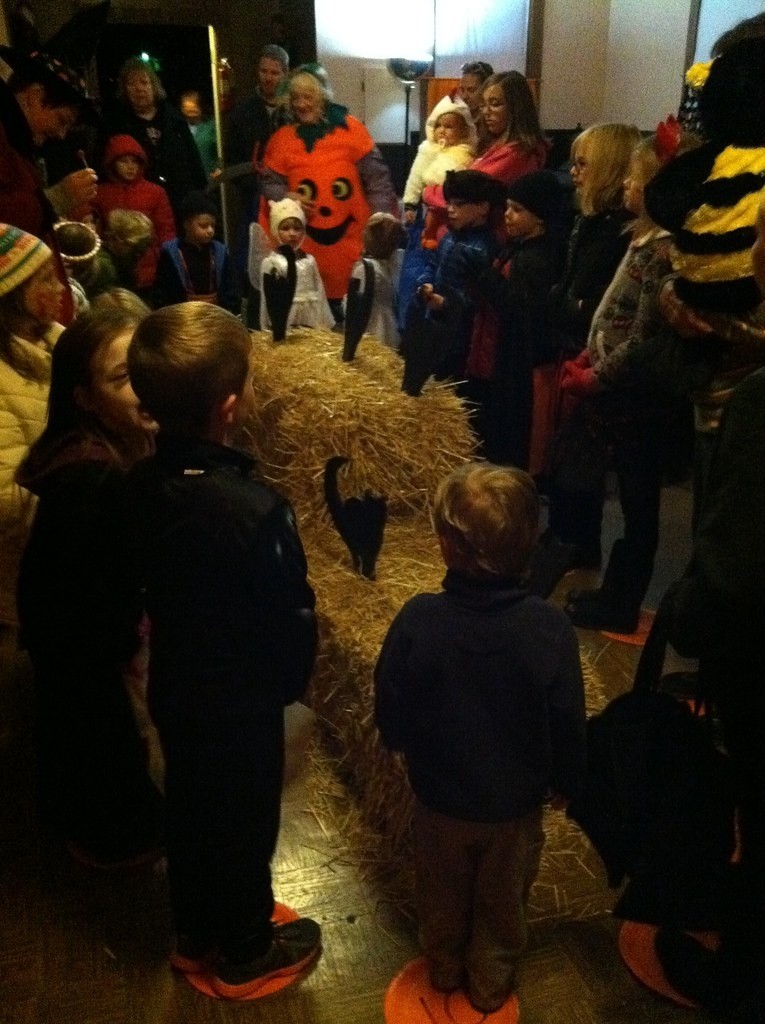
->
[420,76,539,161]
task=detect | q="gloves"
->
[559,351,600,395]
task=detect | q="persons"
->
[0,40,400,343]
[399,14,765,636]
[656,205,765,1024]
[0,222,323,999]
[373,464,589,1014]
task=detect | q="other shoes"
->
[655,926,715,1003]
[537,526,560,548]
[565,586,604,620]
[171,924,208,972]
[212,917,321,999]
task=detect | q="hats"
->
[363,211,405,257]
[0,221,53,296]
[267,198,307,251]
[179,195,218,220]
[506,171,562,222]
[1,44,108,136]
[441,170,504,206]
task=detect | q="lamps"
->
[386,54,433,157]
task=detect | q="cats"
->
[319,454,390,586]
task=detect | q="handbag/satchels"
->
[527,361,584,477]
[556,580,736,897]
